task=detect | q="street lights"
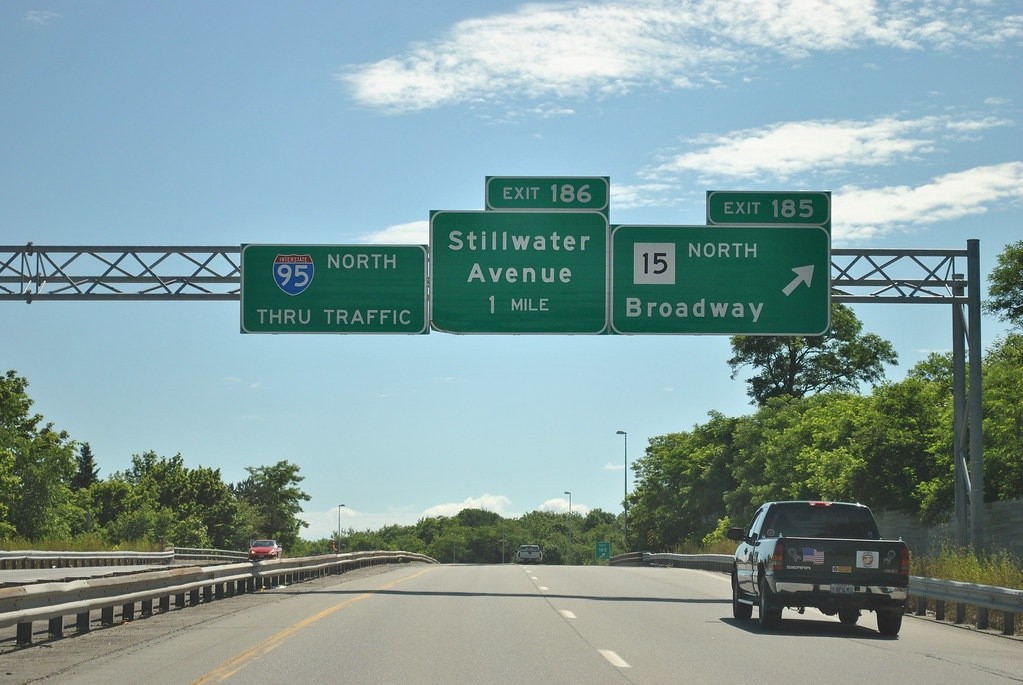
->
[564,491,572,550]
[498,522,505,564]
[616,430,628,553]
[451,533,455,563]
[338,504,346,554]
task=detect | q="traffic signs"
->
[595,542,610,560]
[609,189,834,337]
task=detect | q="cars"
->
[514,544,542,564]
[248,539,284,561]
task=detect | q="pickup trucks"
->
[726,499,910,637]
